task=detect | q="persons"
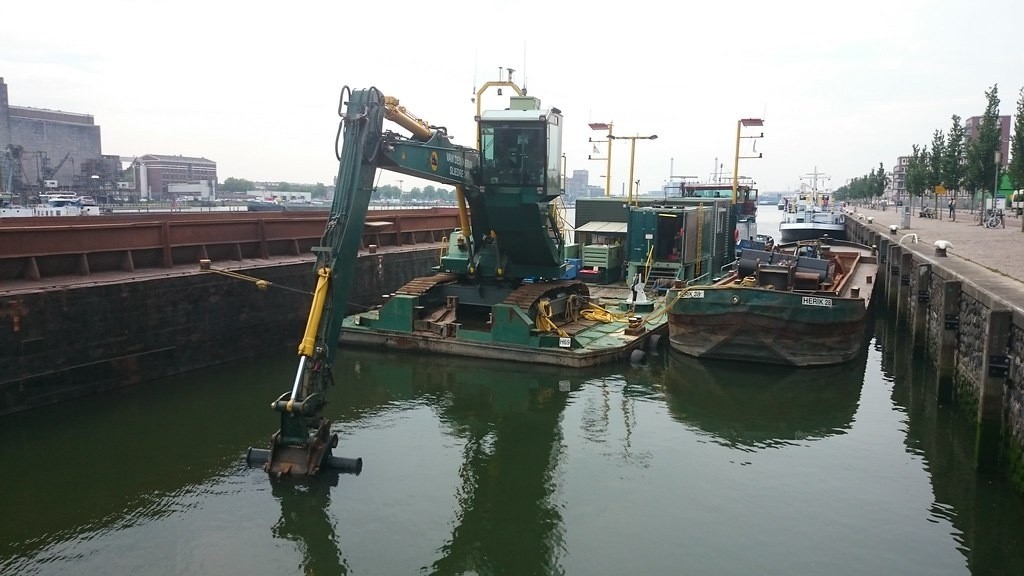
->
[917,204,929,218]
[948,198,953,218]
[673,231,682,261]
[505,135,520,167]
[79,207,90,216]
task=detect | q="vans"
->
[48,194,79,208]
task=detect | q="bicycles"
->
[974,208,1002,229]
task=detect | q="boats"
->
[779,165,848,244]
[664,235,881,368]
[339,117,765,367]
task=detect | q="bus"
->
[1013,189,1024,215]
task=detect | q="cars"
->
[864,200,902,206]
[78,196,96,206]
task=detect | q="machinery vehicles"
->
[241,89,593,481]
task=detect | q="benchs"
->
[921,208,934,218]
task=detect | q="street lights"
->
[606,132,660,208]
[993,151,1001,210]
